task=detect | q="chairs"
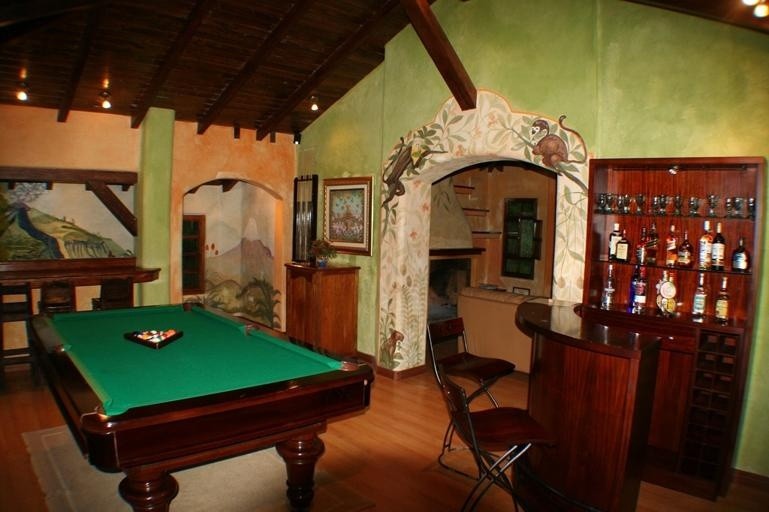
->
[427,317,515,482]
[38,278,77,313]
[92,276,134,310]
[0,282,40,389]
[437,363,560,512]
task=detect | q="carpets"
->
[21,425,334,512]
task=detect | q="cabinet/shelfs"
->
[580,157,767,503]
[430,185,502,260]
[284,262,361,362]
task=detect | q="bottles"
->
[602,263,731,328]
[609,220,751,274]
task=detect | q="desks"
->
[0,257,161,288]
[27,302,375,512]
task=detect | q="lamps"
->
[308,96,319,111]
[97,91,112,109]
[293,130,301,145]
[16,82,30,101]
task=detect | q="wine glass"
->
[596,192,756,219]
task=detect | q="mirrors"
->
[292,174,318,263]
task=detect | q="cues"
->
[299,173,310,258]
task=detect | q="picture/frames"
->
[322,176,372,257]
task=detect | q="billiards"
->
[131,329,176,343]
